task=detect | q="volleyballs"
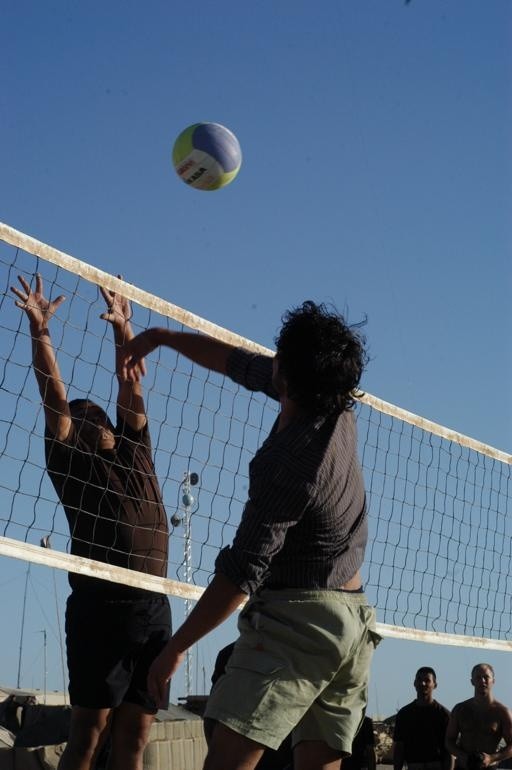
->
[172,121,242,189]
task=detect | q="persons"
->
[4,269,173,769]
[390,666,455,770]
[341,712,376,770]
[448,659,511,769]
[113,298,381,769]
